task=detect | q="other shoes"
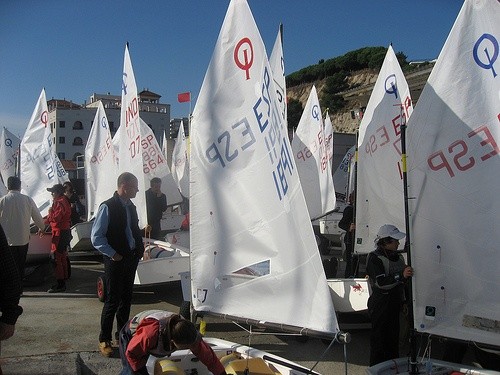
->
[98,340,115,357]
[48,284,67,293]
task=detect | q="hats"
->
[374,224,406,241]
[47,184,66,195]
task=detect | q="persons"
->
[62,181,85,227]
[0,176,46,296]
[365,225,414,367]
[0,223,23,375]
[42,184,73,293]
[142,178,167,240]
[90,173,145,358]
[338,191,356,279]
[118,309,233,375]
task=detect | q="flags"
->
[177,92,190,103]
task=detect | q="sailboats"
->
[145,0,351,375]
[366,1,500,375]
[0,23,413,330]
